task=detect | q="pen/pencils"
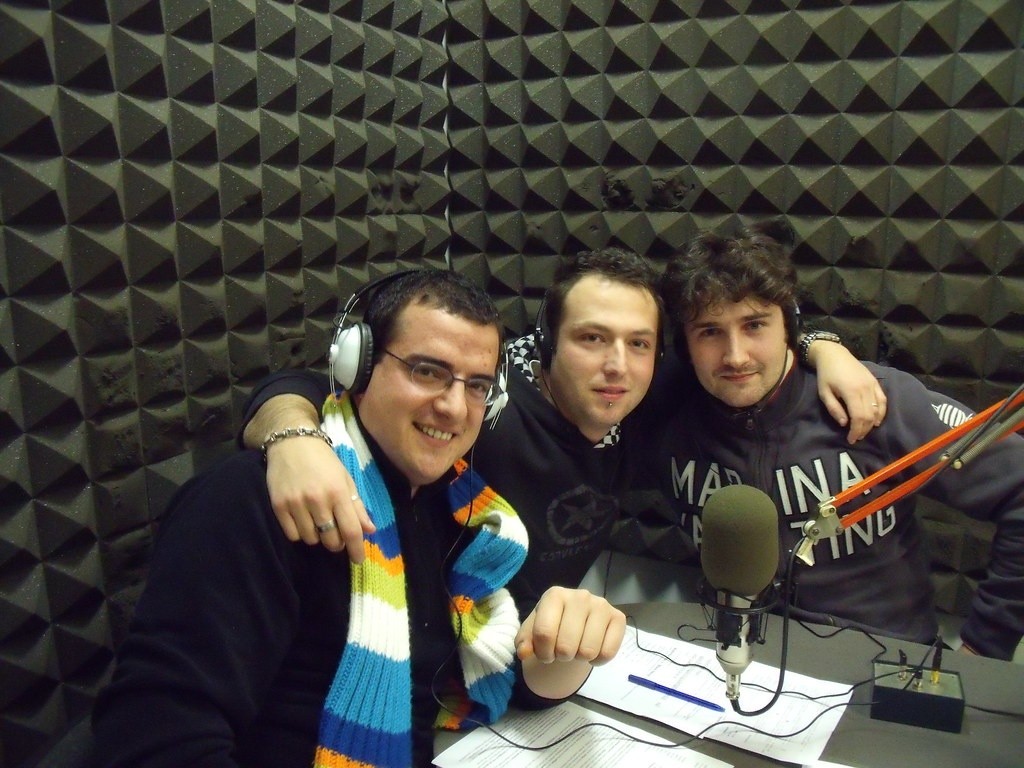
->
[628,674,726,713]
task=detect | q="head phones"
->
[533,280,666,385]
[329,268,509,430]
[670,290,800,377]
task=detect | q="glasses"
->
[372,348,500,404]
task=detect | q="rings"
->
[348,492,362,502]
[313,521,338,535]
[873,400,881,410]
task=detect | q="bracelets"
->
[798,329,845,368]
[257,424,337,468]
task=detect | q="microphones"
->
[702,486,778,700]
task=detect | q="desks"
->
[434,603,1023,768]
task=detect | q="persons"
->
[221,245,892,623]
[613,229,1024,671]
[87,262,633,766]
